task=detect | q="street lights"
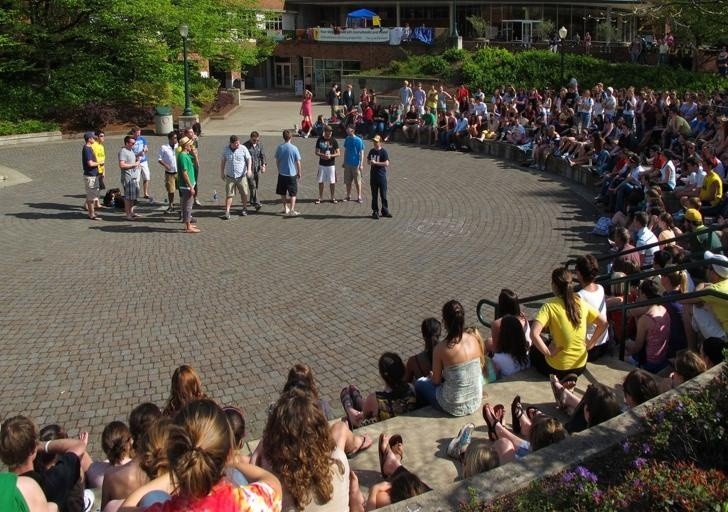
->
[178,21,194,116]
[557,25,569,89]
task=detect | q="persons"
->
[83,125,302,232]
[403,78,728,477]
[292,80,413,218]
[532,29,727,74]
[0,351,433,512]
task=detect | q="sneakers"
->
[372,211,379,219]
[84,489,95,512]
[593,181,602,187]
[610,246,619,253]
[383,212,393,217]
[125,216,134,221]
[255,204,263,210]
[342,197,351,201]
[607,238,614,248]
[183,217,197,223]
[241,207,248,216]
[440,145,445,148]
[594,193,603,200]
[446,145,450,150]
[290,210,301,216]
[446,423,475,458]
[131,212,141,217]
[358,198,363,203]
[284,208,290,213]
[223,213,230,220]
[165,207,174,212]
[315,198,324,204]
[194,201,201,207]
[331,197,338,204]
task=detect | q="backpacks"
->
[592,217,616,237]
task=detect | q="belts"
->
[165,171,177,174]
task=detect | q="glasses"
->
[129,142,135,145]
[99,135,104,137]
[253,139,259,142]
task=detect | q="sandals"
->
[349,383,362,407]
[482,403,501,441]
[494,404,504,424]
[341,387,354,415]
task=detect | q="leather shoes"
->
[185,225,197,230]
[186,228,200,233]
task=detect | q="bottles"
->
[213,190,217,202]
[133,161,141,172]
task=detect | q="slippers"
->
[549,373,565,409]
[378,433,390,477]
[560,373,577,392]
[144,194,149,199]
[346,434,373,459]
[389,435,403,462]
[95,204,106,209]
[526,406,544,419]
[81,205,89,210]
[88,215,104,220]
[511,395,524,433]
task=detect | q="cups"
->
[326,150,330,155]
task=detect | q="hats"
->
[322,125,334,131]
[372,135,382,142]
[608,86,614,93]
[179,136,191,145]
[708,156,717,168]
[84,131,98,141]
[704,250,728,279]
[679,208,703,222]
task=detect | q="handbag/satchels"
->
[448,144,456,150]
[461,143,471,152]
[103,188,121,207]
[114,196,137,208]
[607,319,617,351]
